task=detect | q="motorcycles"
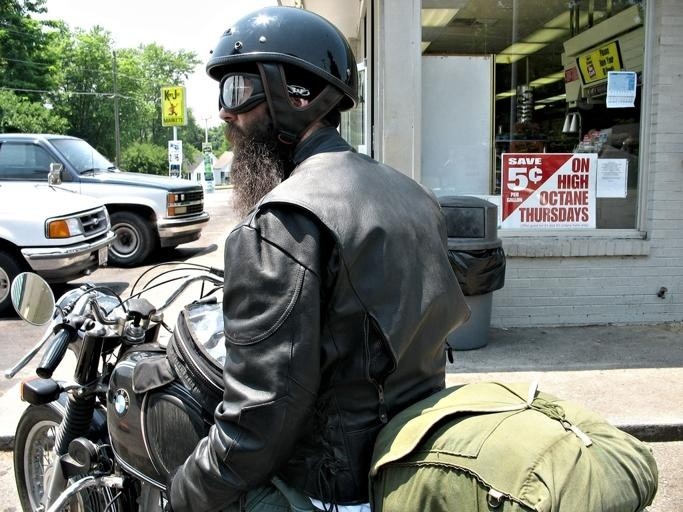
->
[5,261,224,512]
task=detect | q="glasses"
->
[215,69,312,115]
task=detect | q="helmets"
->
[204,4,360,112]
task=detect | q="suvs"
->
[0,133,210,315]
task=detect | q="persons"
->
[160,5,474,512]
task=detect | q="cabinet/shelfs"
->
[494,140,548,193]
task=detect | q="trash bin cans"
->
[437,196,502,351]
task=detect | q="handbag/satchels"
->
[358,376,660,512]
